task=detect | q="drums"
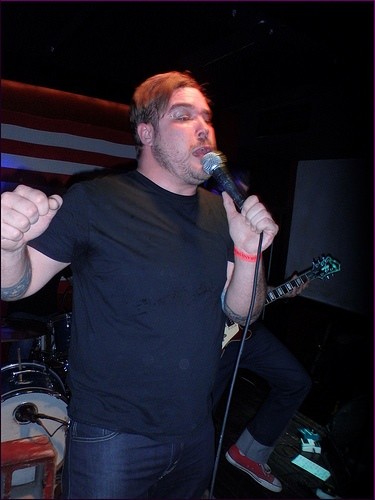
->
[0,363,69,487]
[49,312,72,351]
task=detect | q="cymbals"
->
[0,317,49,343]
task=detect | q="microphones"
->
[201,152,246,211]
[25,407,42,425]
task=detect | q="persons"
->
[209,273,313,493]
[1,72,279,498]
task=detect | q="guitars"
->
[220,255,341,360]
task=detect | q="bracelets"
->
[234,246,262,262]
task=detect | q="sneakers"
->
[226,443,282,492]
